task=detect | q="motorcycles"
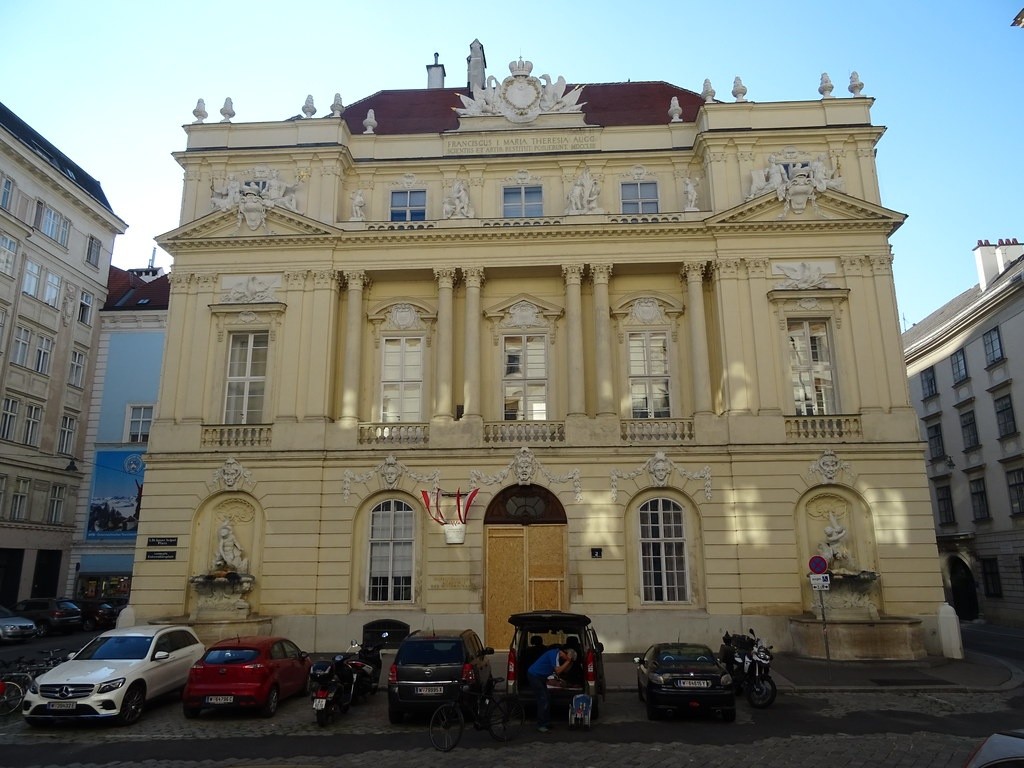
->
[719,628,778,710]
[306,631,388,727]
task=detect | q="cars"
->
[21,624,205,726]
[0,605,37,643]
[633,643,737,724]
[181,634,312,719]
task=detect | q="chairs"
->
[566,636,582,673]
[423,644,435,652]
[662,652,671,660]
[522,635,547,662]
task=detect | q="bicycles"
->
[428,676,526,753]
[0,648,68,717]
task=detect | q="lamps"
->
[64,458,78,472]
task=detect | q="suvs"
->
[387,629,495,726]
[55,598,118,631]
[7,598,82,638]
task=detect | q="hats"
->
[564,648,578,661]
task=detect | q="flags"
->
[422,487,481,525]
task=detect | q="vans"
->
[505,610,606,719]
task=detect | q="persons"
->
[824,525,851,560]
[527,648,579,734]
[650,452,671,480]
[220,458,243,487]
[819,456,836,478]
[763,154,789,190]
[88,502,121,523]
[217,524,249,574]
[514,455,535,480]
[350,189,366,218]
[210,173,241,208]
[260,168,305,217]
[381,455,403,484]
[683,177,700,209]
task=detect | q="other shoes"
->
[535,726,549,732]
